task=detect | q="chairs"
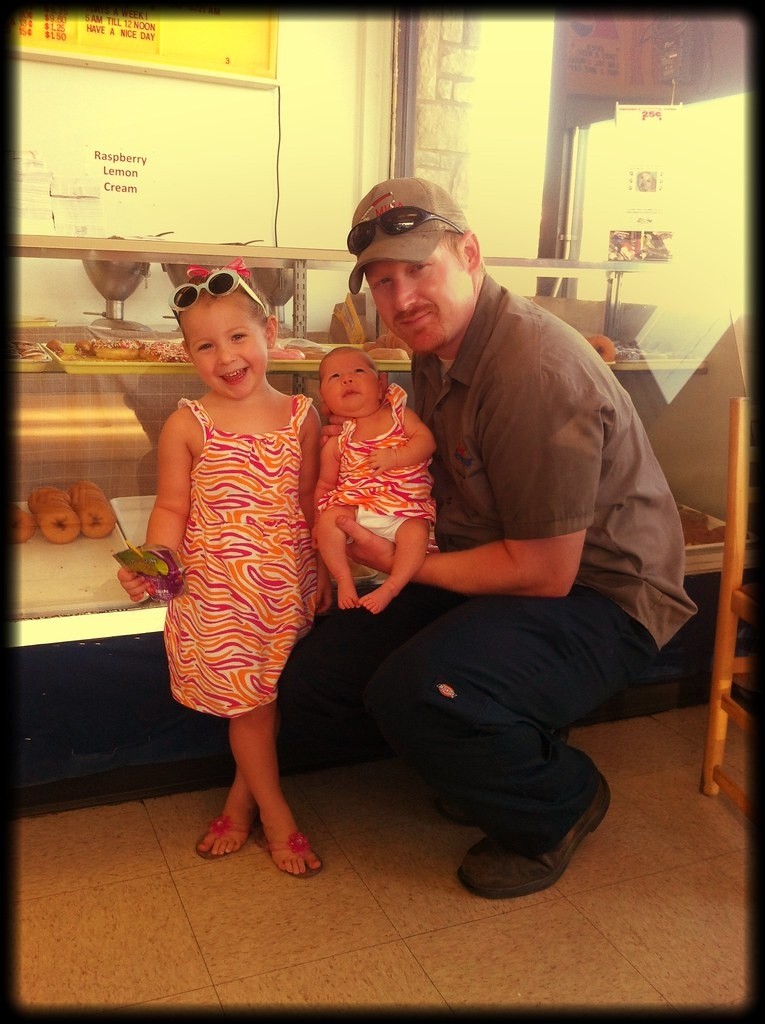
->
[696,396,754,818]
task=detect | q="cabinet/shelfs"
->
[11,232,751,650]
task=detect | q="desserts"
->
[6,481,116,543]
[94,337,191,364]
[4,340,91,360]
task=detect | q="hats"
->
[347,177,469,296]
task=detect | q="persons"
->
[115,263,328,876]
[287,177,700,904]
[310,348,441,613]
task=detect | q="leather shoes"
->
[458,774,610,898]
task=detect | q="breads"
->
[270,329,412,360]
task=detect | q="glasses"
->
[347,205,466,254]
[168,271,267,336]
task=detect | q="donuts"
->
[588,334,616,363]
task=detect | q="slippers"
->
[260,831,322,876]
[192,818,253,861]
